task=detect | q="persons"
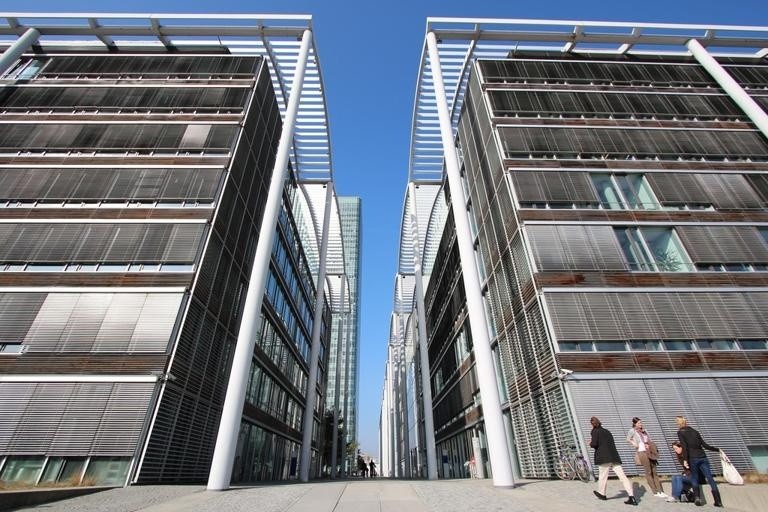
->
[674,415,723,507]
[589,416,638,506]
[663,440,707,503]
[359,460,367,478]
[625,416,669,497]
[368,459,376,478]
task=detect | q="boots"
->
[712,488,722,507]
[693,488,701,505]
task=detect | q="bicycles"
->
[552,441,591,483]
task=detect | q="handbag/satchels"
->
[635,452,643,466]
[682,487,694,502]
[721,459,744,484]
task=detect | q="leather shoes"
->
[624,498,636,505]
[593,491,607,500]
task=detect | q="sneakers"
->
[654,490,669,497]
[666,497,681,502]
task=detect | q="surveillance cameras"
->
[150,371,163,375]
[167,372,177,381]
[560,369,573,374]
[550,371,557,377]
